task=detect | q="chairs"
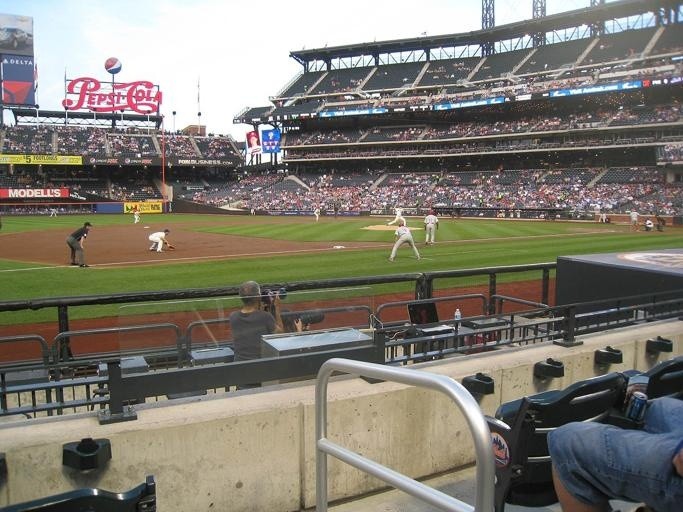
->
[0,473,157,512]
[0,123,240,202]
[483,355,682,512]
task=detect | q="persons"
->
[0,126,244,219]
[546,395,683,512]
[387,222,422,263]
[229,280,285,391]
[148,227,170,253]
[423,210,440,246]
[243,42,682,233]
[64,220,94,268]
[134,210,142,224]
[278,307,311,332]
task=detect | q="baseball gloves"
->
[167,244,175,252]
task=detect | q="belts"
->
[427,222,435,224]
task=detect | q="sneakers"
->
[149,246,165,253]
[71,262,91,267]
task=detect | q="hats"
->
[430,210,436,215]
[399,223,404,227]
[84,221,92,226]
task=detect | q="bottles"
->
[455,309,461,329]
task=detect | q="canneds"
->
[624,392,649,421]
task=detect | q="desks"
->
[187,347,235,394]
[411,317,509,367]
[99,354,148,410]
